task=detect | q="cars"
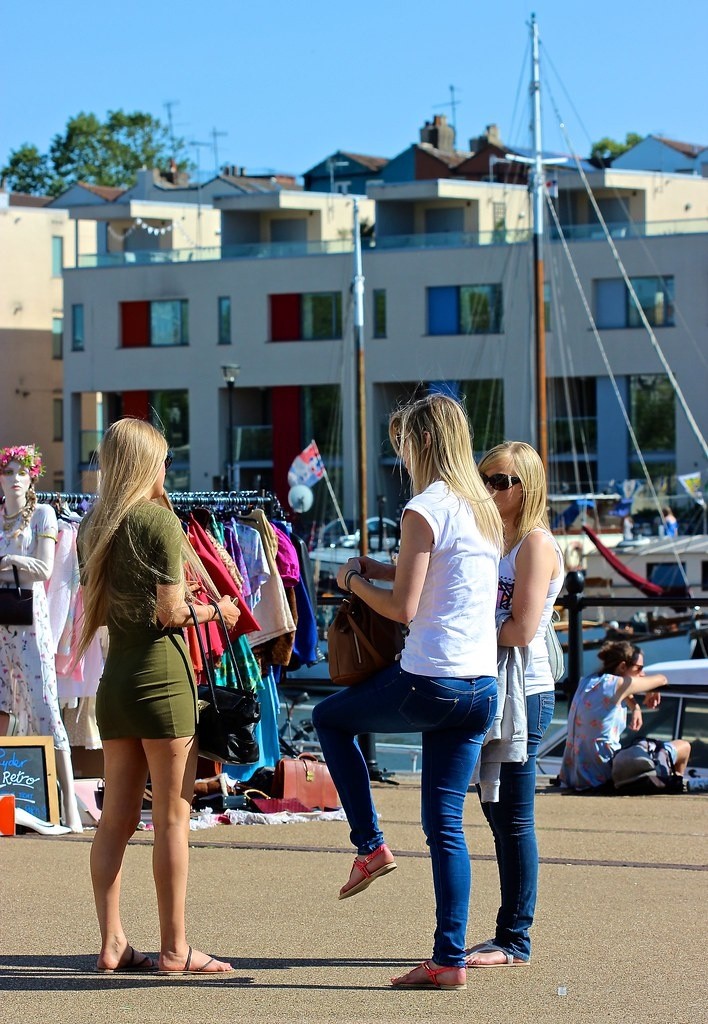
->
[308,516,396,550]
[533,658,708,784]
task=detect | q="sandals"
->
[464,940,530,967]
[386,961,469,989]
[339,844,397,898]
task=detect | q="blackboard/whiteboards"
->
[0,736,59,824]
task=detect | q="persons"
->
[559,640,692,795]
[464,440,566,969]
[312,394,504,988]
[662,506,678,537]
[572,504,595,533]
[76,418,242,974]
[0,444,85,836]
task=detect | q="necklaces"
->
[2,501,30,554]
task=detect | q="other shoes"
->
[15,808,72,837]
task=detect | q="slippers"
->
[155,946,235,975]
[95,945,155,972]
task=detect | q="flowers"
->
[0,442,47,479]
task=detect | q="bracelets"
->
[344,569,363,592]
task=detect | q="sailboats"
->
[302,3,708,685]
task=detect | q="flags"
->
[288,440,327,490]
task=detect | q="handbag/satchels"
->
[328,595,404,685]
[273,754,340,813]
[195,684,262,763]
[244,789,313,813]
[0,553,35,627]
[545,610,565,683]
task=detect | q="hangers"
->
[34,491,265,521]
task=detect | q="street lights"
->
[221,362,243,486]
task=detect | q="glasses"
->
[164,451,174,469]
[478,472,521,491]
[627,662,645,672]
[396,433,406,449]
